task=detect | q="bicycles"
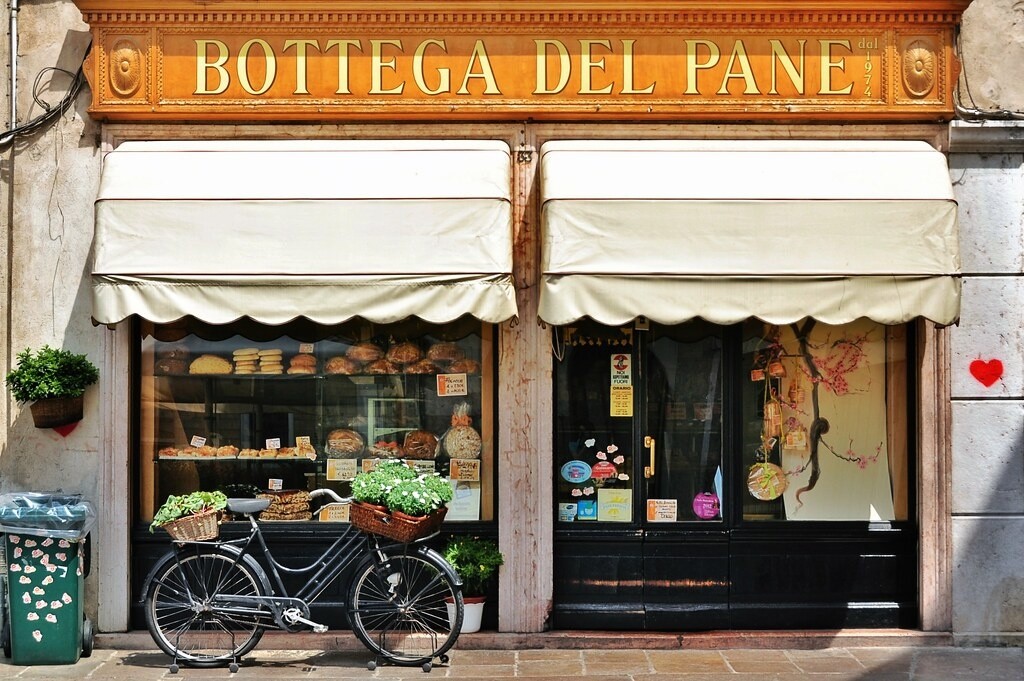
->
[139,472,465,668]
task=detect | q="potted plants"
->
[147,490,228,544]
[4,343,100,427]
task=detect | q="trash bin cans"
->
[0,492,97,667]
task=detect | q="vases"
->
[347,499,448,542]
[444,595,487,635]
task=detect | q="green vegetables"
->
[148,490,227,534]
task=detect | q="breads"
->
[155,330,482,520]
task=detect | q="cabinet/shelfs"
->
[138,316,496,521]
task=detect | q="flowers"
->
[422,535,504,596]
[347,457,454,516]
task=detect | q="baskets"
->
[161,509,219,547]
[350,501,448,543]
[30,390,84,428]
